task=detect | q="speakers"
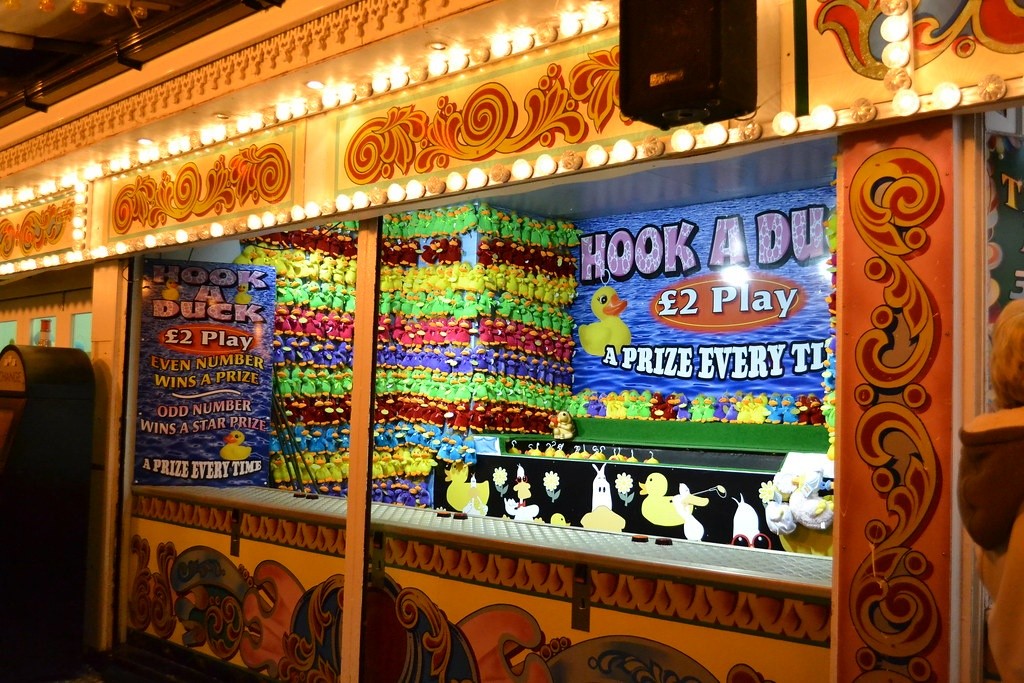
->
[619,0,758,131]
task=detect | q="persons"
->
[956,297,1024,683]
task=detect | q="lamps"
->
[4,0,149,17]
